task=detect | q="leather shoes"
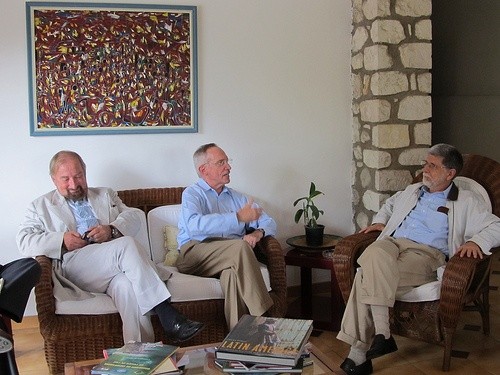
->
[366,334,398,359]
[340,357,373,375]
[164,314,202,339]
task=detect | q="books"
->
[214,315,315,375]
[90,339,189,375]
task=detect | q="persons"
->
[16,151,207,345]
[175,143,284,334]
[336,143,500,375]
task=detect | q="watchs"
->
[110,225,118,239]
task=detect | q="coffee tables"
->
[62,338,348,375]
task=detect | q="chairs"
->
[334,154,500,372]
[0,258,40,375]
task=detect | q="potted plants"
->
[293,182,326,246]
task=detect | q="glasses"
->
[421,159,448,169]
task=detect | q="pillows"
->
[163,224,181,267]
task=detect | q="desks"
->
[281,246,346,332]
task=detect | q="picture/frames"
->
[25,1,198,137]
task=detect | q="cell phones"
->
[81,231,91,240]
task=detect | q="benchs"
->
[31,187,286,375]
